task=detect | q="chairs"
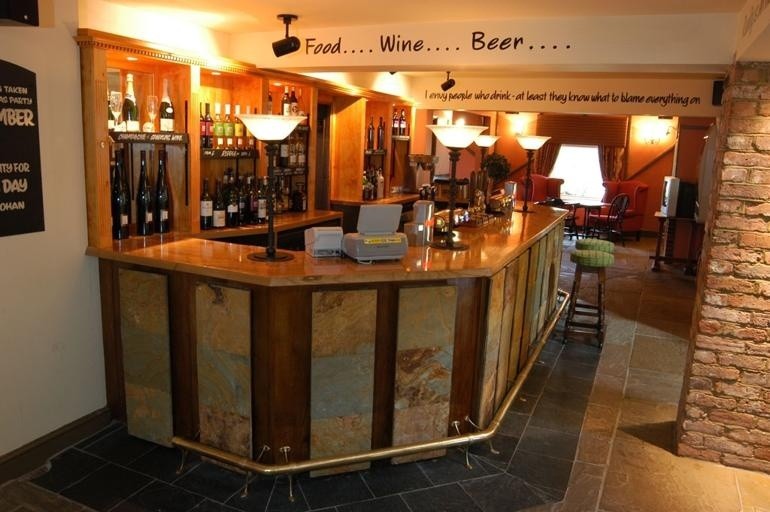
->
[524,174,564,204]
[590,180,648,241]
[588,193,630,247]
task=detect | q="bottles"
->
[399,109,406,135]
[136,150,153,236]
[113,151,130,240]
[200,178,213,231]
[224,175,269,228]
[377,117,385,149]
[298,87,305,116]
[281,85,290,115]
[294,183,306,212]
[367,116,375,150]
[200,102,205,148]
[234,105,244,150]
[272,133,307,168]
[393,108,399,135]
[206,103,214,149]
[363,167,384,200]
[213,178,226,229]
[224,104,234,149]
[244,106,255,150]
[153,150,170,234]
[214,103,224,149]
[290,87,298,116]
[271,178,293,214]
[123,74,137,132]
[160,79,175,133]
[267,91,273,114]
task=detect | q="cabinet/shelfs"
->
[266,78,315,225]
[192,69,265,232]
[330,98,419,206]
[82,49,192,242]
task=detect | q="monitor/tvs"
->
[660,175,699,219]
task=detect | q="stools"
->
[563,250,615,349]
[566,237,615,350]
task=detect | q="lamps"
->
[441,73,455,92]
[517,135,552,212]
[426,124,488,251]
[474,134,501,203]
[273,24,300,57]
[235,113,308,263]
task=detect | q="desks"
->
[559,198,610,240]
[652,211,701,276]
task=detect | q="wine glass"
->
[146,95,159,133]
[110,91,123,132]
